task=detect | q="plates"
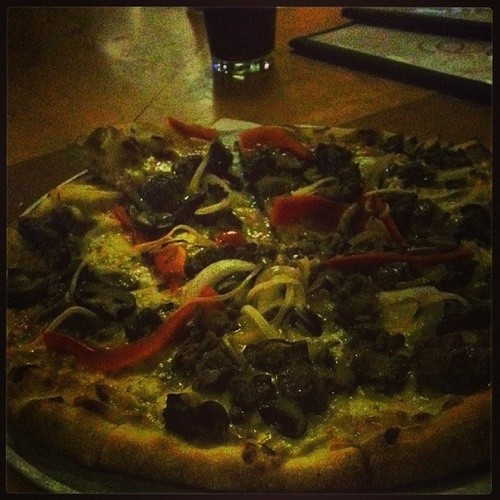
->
[6,125,492,494]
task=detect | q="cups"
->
[203,7,277,75]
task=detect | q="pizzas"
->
[0,120,500,500]
